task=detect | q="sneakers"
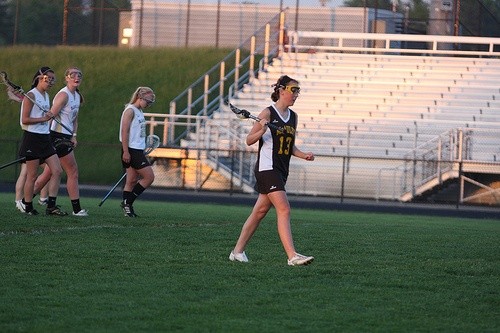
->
[21,199,39,215]
[229,250,248,262]
[288,253,314,266]
[120,199,136,217]
[46,207,66,215]
[73,209,88,216]
[38,199,47,206]
[16,200,26,212]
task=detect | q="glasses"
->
[143,97,153,104]
[44,76,55,83]
[284,85,301,94]
[70,72,82,78]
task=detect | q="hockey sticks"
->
[98,135,160,207]
[0,137,74,169]
[230,103,283,132]
[0,72,77,136]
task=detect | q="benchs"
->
[181,52,500,163]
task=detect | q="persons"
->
[229,75,314,266]
[119,87,155,218]
[20,67,69,217]
[15,146,49,210]
[18,66,89,216]
